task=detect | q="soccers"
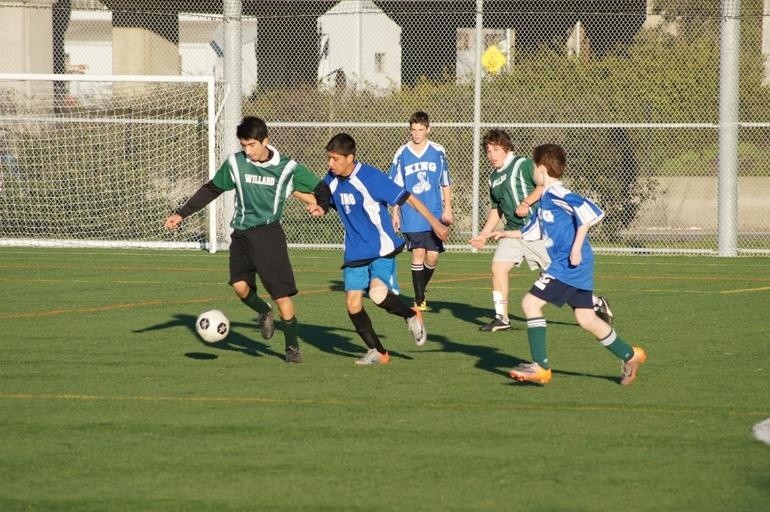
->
[195,308,230,344]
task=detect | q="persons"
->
[163,117,330,363]
[479,145,646,386]
[467,129,614,332]
[387,111,454,311]
[306,134,449,366]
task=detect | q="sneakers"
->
[595,295,614,326]
[405,308,427,348]
[352,346,390,368]
[620,346,646,387]
[284,343,302,363]
[509,361,553,388]
[411,293,429,313]
[478,315,513,334]
[258,306,276,339]
